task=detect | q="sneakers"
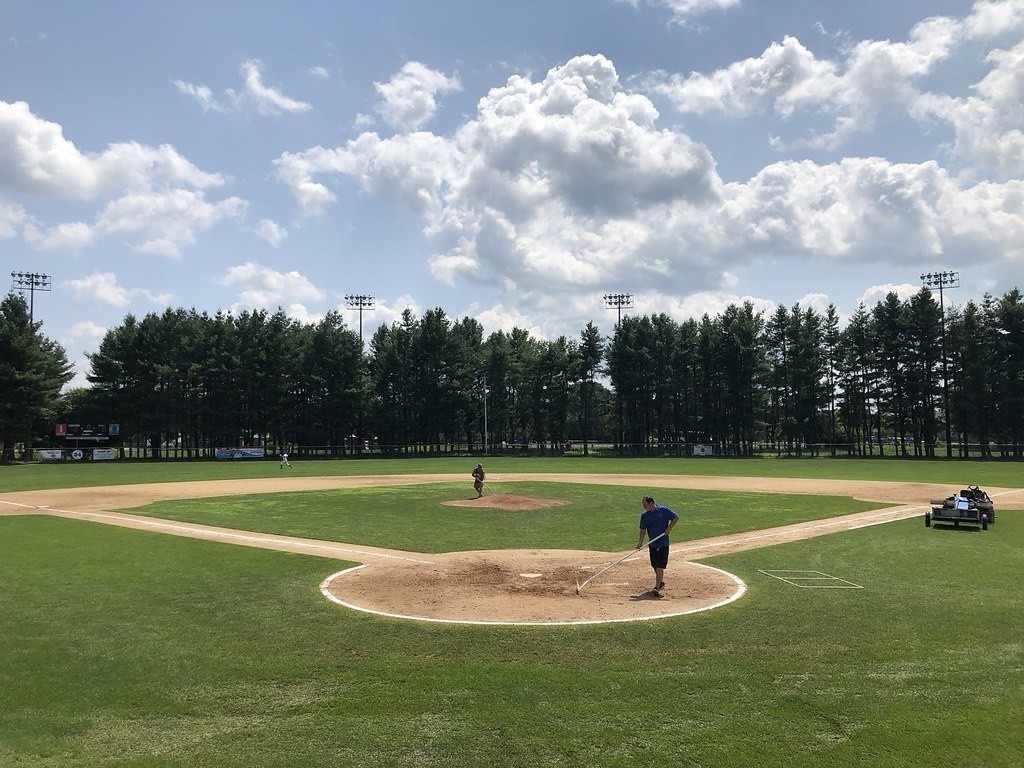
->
[654,582,665,590]
[652,588,659,596]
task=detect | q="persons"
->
[472,463,485,498]
[635,496,679,596]
[279,452,293,469]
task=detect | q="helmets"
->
[478,463,482,468]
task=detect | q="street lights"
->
[344,292,376,454]
[481,373,491,456]
[604,293,636,457]
[10,271,52,332]
[920,270,960,460]
